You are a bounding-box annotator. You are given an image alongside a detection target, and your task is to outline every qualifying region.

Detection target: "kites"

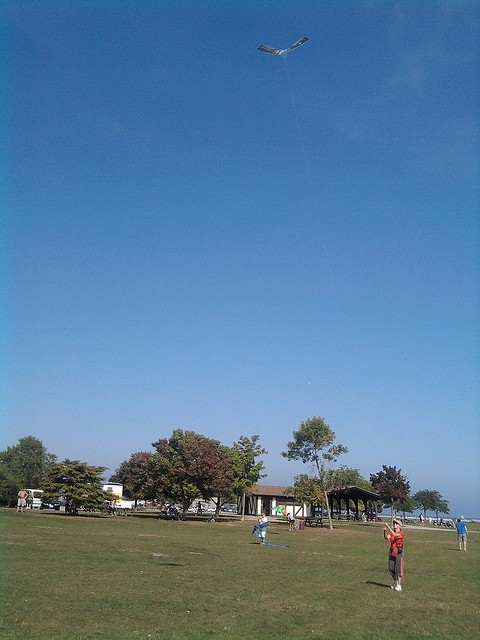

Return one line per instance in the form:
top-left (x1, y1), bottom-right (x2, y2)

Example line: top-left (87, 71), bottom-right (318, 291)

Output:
top-left (256, 37), bottom-right (309, 55)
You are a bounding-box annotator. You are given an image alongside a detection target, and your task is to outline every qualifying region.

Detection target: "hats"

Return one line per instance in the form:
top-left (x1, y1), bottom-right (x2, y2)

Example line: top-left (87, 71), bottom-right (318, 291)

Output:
top-left (393, 518), bottom-right (402, 526)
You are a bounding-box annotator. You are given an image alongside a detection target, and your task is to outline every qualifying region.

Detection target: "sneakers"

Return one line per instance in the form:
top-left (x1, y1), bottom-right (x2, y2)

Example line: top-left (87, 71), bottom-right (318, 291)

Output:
top-left (390, 581), bottom-right (402, 591)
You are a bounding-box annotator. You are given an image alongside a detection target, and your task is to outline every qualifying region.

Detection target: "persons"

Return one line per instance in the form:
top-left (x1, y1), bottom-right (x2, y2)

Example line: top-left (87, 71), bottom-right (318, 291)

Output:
top-left (165, 500), bottom-right (182, 521)
top-left (384, 519), bottom-right (405, 591)
top-left (258, 510), bottom-right (267, 544)
top-left (196, 501), bottom-right (203, 516)
top-left (287, 513), bottom-right (294, 531)
top-left (418, 513), bottom-right (424, 525)
top-left (16, 488), bottom-right (33, 513)
top-left (436, 517), bottom-right (444, 524)
top-left (455, 517), bottom-right (468, 551)
top-left (112, 499), bottom-right (117, 516)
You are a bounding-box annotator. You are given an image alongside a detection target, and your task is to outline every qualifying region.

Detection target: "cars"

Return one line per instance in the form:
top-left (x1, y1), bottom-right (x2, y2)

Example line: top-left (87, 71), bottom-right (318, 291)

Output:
top-left (40, 501), bottom-right (61, 511)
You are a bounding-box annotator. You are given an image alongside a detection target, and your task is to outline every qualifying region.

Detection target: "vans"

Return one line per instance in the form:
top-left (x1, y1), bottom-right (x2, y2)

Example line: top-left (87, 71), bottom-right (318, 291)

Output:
top-left (21, 488), bottom-right (44, 510)
